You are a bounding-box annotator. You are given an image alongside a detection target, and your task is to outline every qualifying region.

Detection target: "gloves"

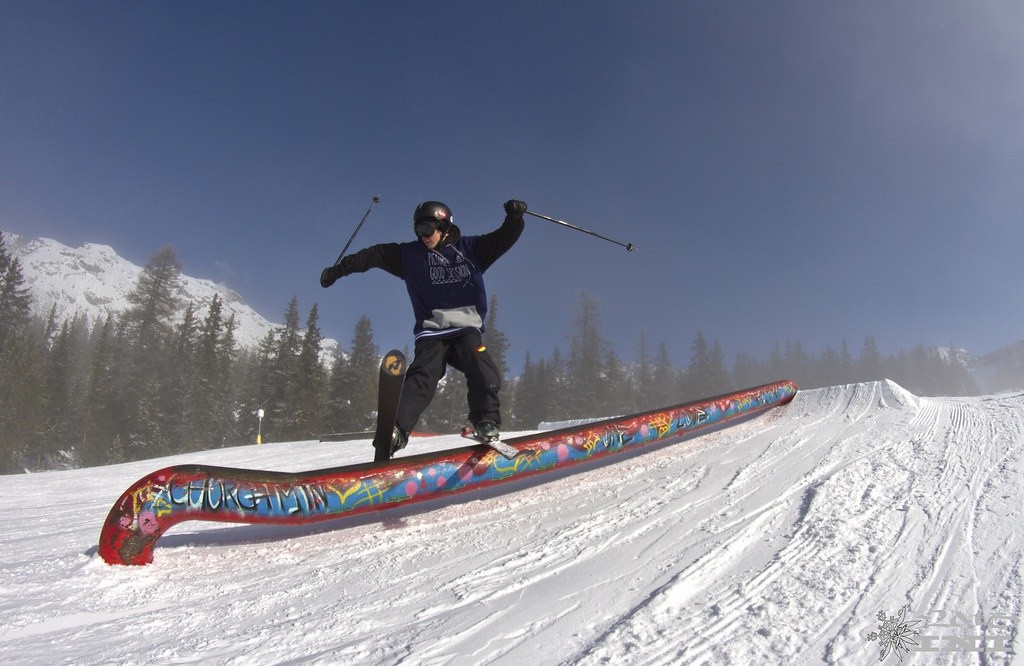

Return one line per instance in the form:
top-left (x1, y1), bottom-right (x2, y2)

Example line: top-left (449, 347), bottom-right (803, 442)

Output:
top-left (320, 266), bottom-right (337, 288)
top-left (505, 199), bottom-right (527, 219)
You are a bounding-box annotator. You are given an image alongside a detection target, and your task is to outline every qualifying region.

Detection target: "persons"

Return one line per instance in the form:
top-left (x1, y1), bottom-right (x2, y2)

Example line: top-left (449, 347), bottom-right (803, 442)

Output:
top-left (320, 198), bottom-right (528, 456)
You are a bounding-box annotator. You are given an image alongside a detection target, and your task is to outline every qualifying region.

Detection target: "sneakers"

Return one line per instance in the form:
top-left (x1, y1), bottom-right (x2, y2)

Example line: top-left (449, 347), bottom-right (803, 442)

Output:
top-left (476, 421), bottom-right (500, 439)
top-left (372, 426), bottom-right (407, 457)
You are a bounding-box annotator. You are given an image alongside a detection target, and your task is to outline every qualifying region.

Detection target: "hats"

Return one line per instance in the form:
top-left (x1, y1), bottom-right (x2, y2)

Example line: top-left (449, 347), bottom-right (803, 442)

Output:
top-left (412, 200), bottom-right (454, 224)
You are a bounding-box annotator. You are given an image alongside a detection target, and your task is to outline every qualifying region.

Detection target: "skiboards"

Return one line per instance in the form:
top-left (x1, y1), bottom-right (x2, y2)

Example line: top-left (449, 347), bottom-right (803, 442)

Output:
top-left (374, 349), bottom-right (408, 462)
top-left (459, 427), bottom-right (520, 460)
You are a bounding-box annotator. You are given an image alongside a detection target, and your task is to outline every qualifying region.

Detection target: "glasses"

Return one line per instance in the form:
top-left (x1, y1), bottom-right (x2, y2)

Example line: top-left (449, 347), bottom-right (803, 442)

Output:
top-left (414, 221), bottom-right (442, 238)
